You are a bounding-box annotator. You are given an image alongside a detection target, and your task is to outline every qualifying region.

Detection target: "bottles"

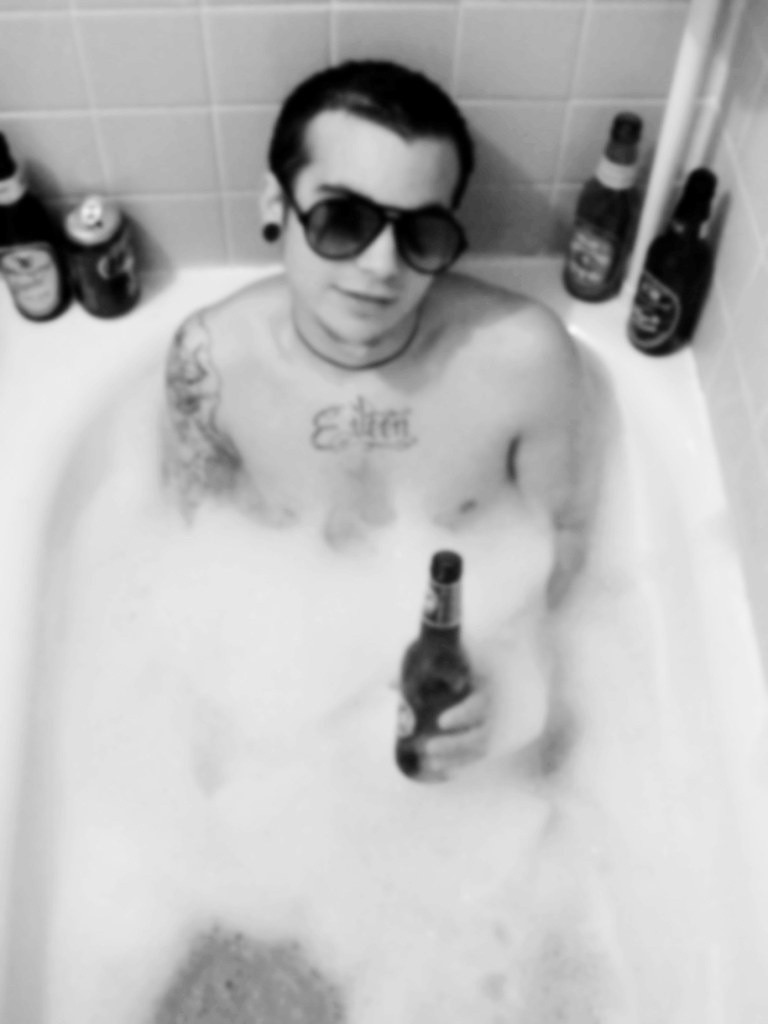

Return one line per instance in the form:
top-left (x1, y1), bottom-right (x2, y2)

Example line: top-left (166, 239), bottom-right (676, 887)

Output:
top-left (627, 166), bottom-right (721, 355)
top-left (398, 549), bottom-right (473, 784)
top-left (562, 110), bottom-right (643, 302)
top-left (0, 130), bottom-right (77, 322)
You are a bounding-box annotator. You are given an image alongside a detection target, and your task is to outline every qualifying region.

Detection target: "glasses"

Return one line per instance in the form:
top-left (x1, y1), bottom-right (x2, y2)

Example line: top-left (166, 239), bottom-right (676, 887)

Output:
top-left (286, 185), bottom-right (468, 274)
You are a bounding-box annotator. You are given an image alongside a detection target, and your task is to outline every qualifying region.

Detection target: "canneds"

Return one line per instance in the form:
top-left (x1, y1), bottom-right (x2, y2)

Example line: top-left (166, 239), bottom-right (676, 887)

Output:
top-left (64, 196), bottom-right (140, 319)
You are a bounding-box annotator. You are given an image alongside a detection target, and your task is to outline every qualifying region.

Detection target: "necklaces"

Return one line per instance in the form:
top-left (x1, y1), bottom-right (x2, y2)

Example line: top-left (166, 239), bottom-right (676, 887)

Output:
top-left (293, 291), bottom-right (424, 371)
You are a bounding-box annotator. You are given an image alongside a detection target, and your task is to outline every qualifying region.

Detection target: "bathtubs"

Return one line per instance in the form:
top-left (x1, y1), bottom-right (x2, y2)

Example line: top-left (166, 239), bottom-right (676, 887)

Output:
top-left (0, 258), bottom-right (767, 1023)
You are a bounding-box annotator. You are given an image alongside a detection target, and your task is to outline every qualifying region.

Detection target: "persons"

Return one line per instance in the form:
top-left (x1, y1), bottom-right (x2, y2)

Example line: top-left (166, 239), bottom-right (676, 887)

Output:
top-left (162, 60), bottom-right (580, 1022)
